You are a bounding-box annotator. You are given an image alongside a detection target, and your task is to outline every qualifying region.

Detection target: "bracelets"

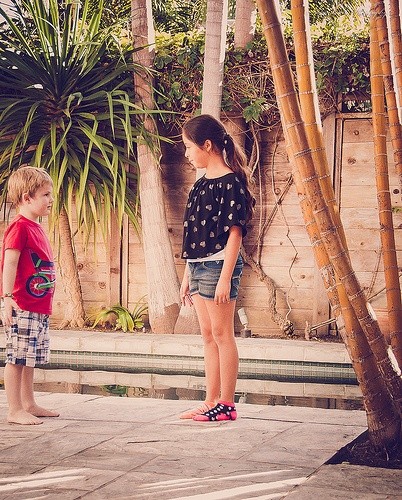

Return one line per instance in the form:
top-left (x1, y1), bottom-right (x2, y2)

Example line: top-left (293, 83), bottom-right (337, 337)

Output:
top-left (2, 292), bottom-right (13, 297)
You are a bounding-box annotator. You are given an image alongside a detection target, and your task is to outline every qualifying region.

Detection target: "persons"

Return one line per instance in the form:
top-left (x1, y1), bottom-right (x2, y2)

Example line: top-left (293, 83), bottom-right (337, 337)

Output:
top-left (176, 114), bottom-right (256, 422)
top-left (0, 164), bottom-right (61, 427)
